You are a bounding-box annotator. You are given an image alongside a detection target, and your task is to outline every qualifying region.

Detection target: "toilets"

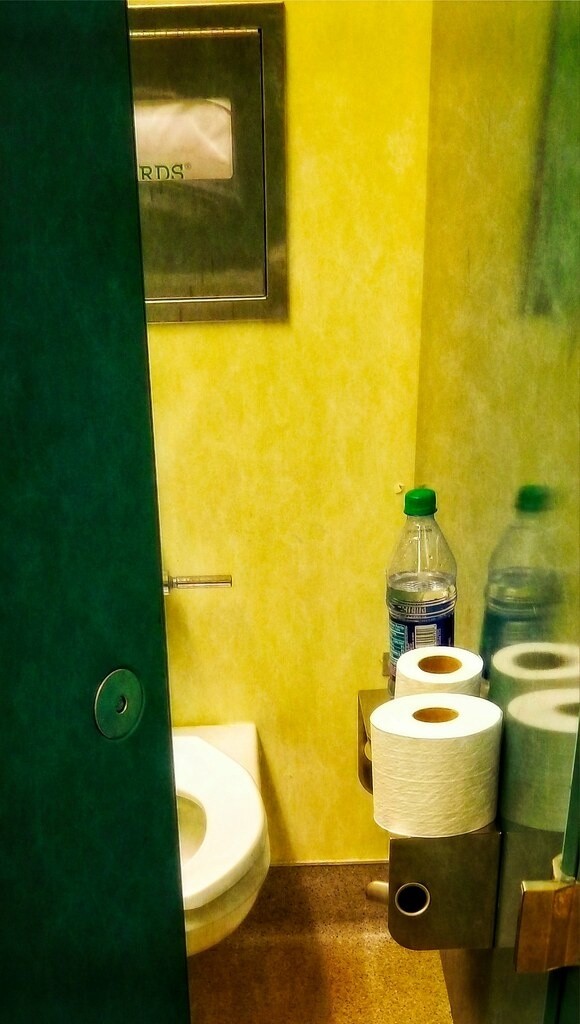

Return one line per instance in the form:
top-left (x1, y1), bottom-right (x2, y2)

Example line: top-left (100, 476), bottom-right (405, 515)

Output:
top-left (173, 721), bottom-right (268, 960)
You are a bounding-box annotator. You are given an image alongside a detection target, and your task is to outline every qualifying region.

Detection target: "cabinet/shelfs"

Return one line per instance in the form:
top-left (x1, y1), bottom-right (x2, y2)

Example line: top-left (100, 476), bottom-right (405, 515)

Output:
top-left (128, 28), bottom-right (267, 302)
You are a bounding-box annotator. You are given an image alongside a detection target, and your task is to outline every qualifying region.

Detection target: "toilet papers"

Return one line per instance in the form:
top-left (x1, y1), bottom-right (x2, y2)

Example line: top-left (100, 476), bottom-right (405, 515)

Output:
top-left (393, 642), bottom-right (486, 706)
top-left (503, 688), bottom-right (580, 833)
top-left (490, 640), bottom-right (580, 711)
top-left (369, 694), bottom-right (502, 840)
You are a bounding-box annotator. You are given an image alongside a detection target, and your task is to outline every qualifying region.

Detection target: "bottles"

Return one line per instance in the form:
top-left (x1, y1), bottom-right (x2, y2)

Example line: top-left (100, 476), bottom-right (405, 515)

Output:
top-left (384, 488), bottom-right (458, 700)
top-left (478, 483), bottom-right (566, 700)
